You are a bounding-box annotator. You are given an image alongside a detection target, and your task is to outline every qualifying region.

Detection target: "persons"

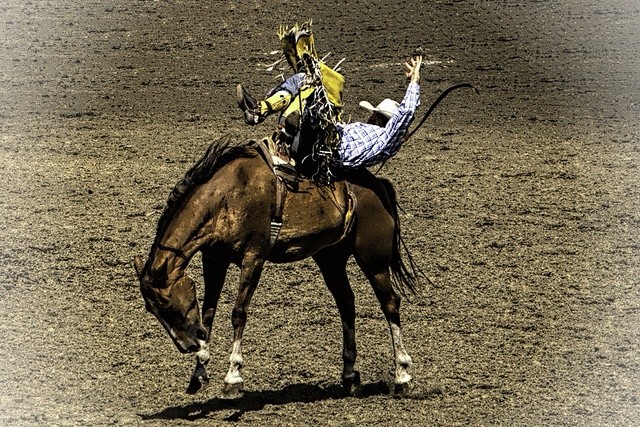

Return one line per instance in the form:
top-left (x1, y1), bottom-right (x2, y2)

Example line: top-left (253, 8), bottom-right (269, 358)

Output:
top-left (236, 53), bottom-right (422, 178)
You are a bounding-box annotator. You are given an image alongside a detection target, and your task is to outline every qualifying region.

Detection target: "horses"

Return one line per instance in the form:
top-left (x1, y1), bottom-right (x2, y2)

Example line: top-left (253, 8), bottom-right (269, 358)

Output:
top-left (134, 132), bottom-right (445, 398)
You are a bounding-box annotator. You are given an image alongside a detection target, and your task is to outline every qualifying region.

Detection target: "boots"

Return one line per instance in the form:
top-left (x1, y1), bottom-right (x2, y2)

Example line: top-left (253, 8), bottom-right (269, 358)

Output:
top-left (278, 86), bottom-right (313, 142)
top-left (237, 84), bottom-right (292, 126)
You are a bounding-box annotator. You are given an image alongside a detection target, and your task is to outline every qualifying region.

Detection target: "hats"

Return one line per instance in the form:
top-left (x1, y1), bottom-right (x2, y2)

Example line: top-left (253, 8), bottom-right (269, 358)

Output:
top-left (359, 99), bottom-right (401, 120)
